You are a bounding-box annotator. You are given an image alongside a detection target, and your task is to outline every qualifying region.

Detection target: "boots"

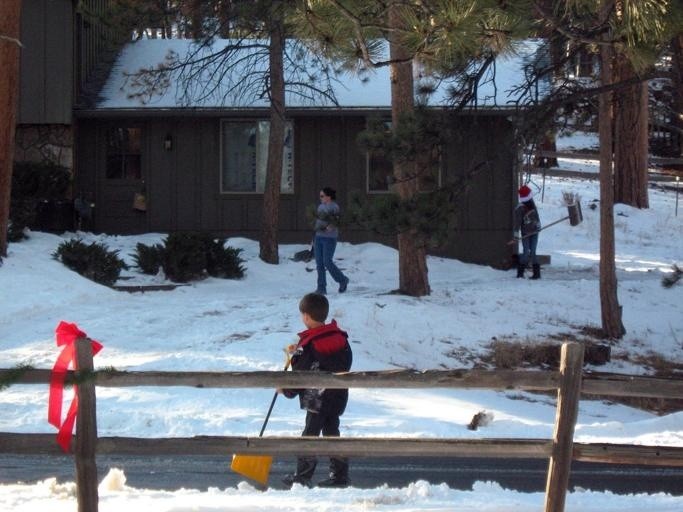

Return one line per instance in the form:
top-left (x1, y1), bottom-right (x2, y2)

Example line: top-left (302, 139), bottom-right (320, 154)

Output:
top-left (529, 263), bottom-right (541, 279)
top-left (517, 263), bottom-right (525, 278)
top-left (319, 454), bottom-right (350, 488)
top-left (282, 456), bottom-right (318, 489)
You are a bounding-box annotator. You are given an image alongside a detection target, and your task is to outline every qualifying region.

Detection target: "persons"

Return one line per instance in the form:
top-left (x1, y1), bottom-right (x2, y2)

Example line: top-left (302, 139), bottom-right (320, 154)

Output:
top-left (509, 183), bottom-right (542, 281)
top-left (306, 186), bottom-right (350, 294)
top-left (274, 291), bottom-right (352, 489)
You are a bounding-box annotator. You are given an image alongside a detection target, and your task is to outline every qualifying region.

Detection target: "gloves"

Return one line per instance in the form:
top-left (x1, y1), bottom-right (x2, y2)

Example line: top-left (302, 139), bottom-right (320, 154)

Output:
top-left (514, 232), bottom-right (519, 240)
top-left (536, 222), bottom-right (540, 231)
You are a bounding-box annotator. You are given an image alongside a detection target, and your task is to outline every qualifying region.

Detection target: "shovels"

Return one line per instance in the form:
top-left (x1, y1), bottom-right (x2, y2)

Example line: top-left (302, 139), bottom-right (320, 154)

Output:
top-left (507, 201), bottom-right (583, 246)
top-left (230, 345), bottom-right (297, 485)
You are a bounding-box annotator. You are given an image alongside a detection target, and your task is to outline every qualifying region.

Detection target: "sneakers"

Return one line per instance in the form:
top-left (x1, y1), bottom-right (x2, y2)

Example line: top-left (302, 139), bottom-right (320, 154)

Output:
top-left (313, 289), bottom-right (327, 295)
top-left (339, 277), bottom-right (348, 293)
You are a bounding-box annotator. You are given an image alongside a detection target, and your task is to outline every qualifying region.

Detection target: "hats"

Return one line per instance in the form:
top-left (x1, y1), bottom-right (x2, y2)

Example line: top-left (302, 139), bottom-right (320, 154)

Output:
top-left (518, 186), bottom-right (532, 202)
